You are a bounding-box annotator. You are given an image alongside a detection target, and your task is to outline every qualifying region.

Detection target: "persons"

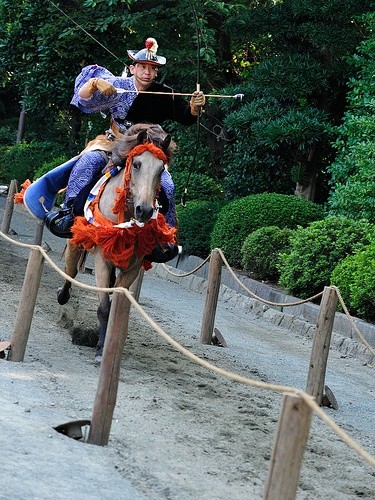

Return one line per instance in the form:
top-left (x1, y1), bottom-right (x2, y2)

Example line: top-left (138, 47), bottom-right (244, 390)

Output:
top-left (53, 38), bottom-right (205, 228)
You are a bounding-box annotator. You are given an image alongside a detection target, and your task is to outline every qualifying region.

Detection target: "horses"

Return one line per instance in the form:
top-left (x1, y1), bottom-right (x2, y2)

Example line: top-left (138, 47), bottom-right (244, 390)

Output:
top-left (56, 121), bottom-right (175, 366)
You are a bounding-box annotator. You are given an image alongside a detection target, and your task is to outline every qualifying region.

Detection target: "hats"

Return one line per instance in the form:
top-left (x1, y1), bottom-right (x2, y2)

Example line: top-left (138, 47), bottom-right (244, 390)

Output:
top-left (126, 38), bottom-right (168, 66)
